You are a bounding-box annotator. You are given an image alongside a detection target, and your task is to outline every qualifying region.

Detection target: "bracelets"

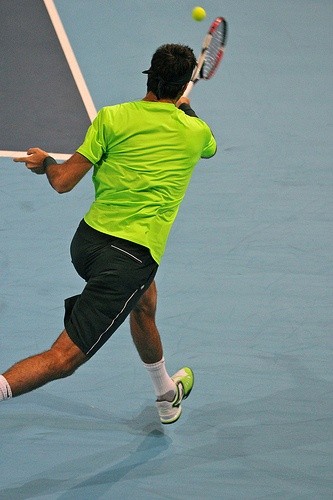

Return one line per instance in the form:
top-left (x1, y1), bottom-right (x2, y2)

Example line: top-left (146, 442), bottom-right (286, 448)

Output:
top-left (42, 157), bottom-right (56, 171)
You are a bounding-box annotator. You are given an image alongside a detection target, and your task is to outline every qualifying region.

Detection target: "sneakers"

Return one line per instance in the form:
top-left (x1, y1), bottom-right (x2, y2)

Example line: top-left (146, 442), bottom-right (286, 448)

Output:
top-left (155, 367), bottom-right (194, 425)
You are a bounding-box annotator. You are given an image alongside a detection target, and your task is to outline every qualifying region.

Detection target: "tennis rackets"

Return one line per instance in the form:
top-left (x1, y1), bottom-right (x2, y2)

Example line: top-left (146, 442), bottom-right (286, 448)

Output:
top-left (182, 16), bottom-right (228, 97)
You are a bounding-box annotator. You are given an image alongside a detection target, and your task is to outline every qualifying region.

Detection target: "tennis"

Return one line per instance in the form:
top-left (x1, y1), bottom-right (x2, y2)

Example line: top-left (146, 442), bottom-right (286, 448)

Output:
top-left (191, 6), bottom-right (207, 22)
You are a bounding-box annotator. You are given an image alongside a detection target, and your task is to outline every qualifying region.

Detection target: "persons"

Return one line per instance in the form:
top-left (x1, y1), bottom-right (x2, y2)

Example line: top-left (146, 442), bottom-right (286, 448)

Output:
top-left (0, 43), bottom-right (218, 425)
